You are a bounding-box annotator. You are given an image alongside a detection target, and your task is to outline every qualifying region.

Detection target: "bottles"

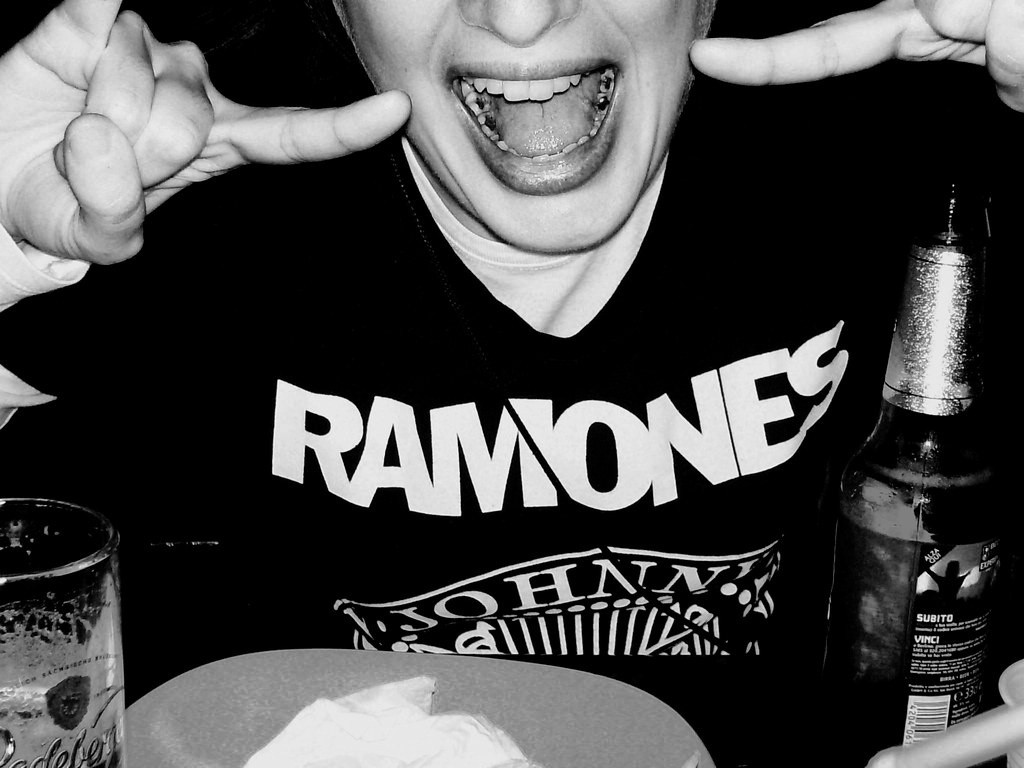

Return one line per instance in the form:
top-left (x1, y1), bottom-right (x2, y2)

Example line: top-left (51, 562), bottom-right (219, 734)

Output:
top-left (817, 173), bottom-right (1002, 768)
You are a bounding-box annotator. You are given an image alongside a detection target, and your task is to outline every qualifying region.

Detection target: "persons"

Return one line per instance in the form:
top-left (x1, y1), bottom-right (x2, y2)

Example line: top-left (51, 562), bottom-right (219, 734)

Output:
top-left (0, 0), bottom-right (1024, 656)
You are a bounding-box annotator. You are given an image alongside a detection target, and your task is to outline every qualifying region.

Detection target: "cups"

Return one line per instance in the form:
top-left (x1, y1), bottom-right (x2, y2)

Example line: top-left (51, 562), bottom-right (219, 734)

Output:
top-left (0, 499), bottom-right (128, 768)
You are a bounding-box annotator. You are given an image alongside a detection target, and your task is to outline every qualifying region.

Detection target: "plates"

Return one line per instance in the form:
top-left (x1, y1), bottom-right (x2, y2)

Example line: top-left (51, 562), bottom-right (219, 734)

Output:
top-left (121, 649), bottom-right (715, 768)
